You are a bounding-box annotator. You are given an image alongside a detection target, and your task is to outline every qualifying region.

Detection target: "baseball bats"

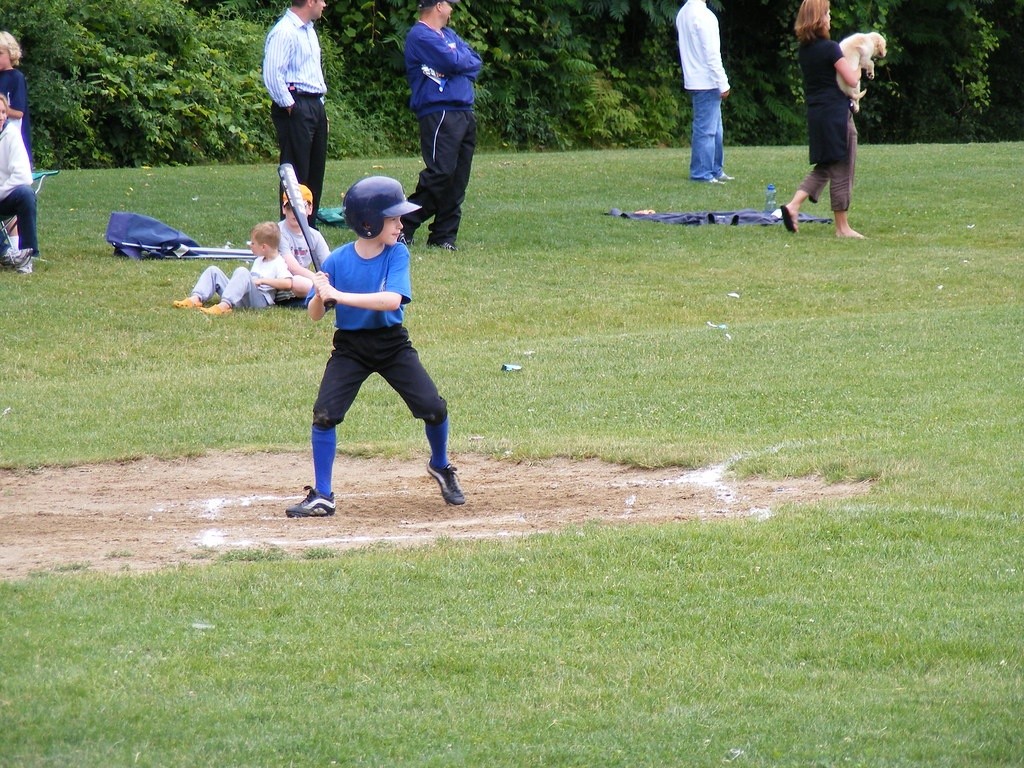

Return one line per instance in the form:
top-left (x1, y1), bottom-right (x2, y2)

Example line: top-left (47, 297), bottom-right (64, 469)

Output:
top-left (277, 163), bottom-right (337, 308)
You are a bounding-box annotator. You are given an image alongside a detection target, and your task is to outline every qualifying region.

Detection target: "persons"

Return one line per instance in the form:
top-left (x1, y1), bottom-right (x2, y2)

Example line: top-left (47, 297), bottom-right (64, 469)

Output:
top-left (0, 92), bottom-right (39, 274)
top-left (0, 31), bottom-right (31, 251)
top-left (780, 0), bottom-right (864, 238)
top-left (263, 0), bottom-right (327, 229)
top-left (285, 176), bottom-right (465, 518)
top-left (173, 221), bottom-right (293, 315)
top-left (397, 0), bottom-right (482, 251)
top-left (676, 0), bottom-right (735, 185)
top-left (0, 233), bottom-right (33, 267)
top-left (277, 184), bottom-right (331, 307)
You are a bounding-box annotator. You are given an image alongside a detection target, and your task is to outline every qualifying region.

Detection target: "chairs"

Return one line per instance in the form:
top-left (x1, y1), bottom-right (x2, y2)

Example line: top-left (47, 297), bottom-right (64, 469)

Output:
top-left (0, 170), bottom-right (60, 251)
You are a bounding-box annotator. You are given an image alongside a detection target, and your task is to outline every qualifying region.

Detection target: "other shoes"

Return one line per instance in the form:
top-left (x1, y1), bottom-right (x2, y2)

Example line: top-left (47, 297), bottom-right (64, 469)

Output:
top-left (398, 230), bottom-right (415, 247)
top-left (438, 240), bottom-right (458, 252)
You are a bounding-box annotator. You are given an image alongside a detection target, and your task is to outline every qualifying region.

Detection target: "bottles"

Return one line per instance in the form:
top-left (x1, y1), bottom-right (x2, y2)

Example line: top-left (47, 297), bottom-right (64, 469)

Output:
top-left (764, 183), bottom-right (777, 213)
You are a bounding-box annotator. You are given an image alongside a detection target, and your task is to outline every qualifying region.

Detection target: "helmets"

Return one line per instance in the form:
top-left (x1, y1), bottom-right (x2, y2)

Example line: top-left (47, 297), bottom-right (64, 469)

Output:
top-left (343, 175), bottom-right (423, 239)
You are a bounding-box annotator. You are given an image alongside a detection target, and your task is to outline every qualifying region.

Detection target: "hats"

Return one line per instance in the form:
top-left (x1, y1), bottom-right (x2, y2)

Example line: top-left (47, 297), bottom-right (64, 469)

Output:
top-left (282, 184), bottom-right (313, 207)
top-left (417, 0), bottom-right (460, 8)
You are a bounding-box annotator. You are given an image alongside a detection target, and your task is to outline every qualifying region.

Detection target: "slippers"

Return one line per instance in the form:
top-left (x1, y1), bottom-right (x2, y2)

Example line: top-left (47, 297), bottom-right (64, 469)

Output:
top-left (779, 205), bottom-right (800, 233)
top-left (198, 304), bottom-right (232, 318)
top-left (172, 298), bottom-right (204, 310)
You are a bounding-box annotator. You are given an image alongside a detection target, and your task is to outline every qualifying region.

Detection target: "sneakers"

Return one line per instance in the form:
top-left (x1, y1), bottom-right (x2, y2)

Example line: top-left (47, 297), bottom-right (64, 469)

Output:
top-left (709, 177), bottom-right (725, 184)
top-left (719, 171), bottom-right (735, 182)
top-left (17, 259), bottom-right (32, 273)
top-left (427, 456), bottom-right (466, 506)
top-left (0, 247), bottom-right (33, 268)
top-left (286, 486), bottom-right (336, 518)
